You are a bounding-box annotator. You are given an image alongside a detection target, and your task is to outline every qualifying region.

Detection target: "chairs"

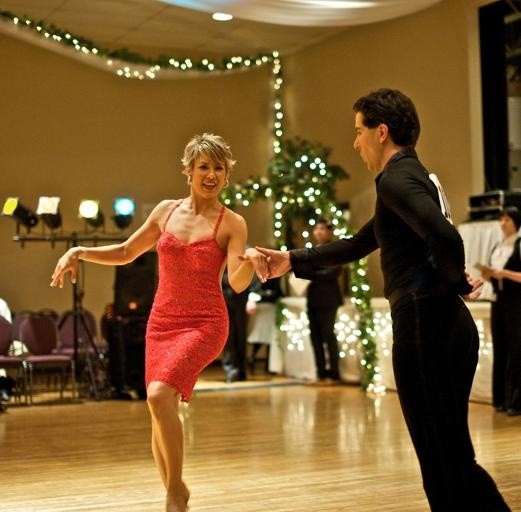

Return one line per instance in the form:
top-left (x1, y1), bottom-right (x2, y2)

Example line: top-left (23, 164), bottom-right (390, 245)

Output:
top-left (0, 309), bottom-right (107, 405)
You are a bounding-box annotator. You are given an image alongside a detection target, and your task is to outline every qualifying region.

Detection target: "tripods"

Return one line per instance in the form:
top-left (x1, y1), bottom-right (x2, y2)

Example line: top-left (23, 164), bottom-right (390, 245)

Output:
top-left (54, 239), bottom-right (113, 401)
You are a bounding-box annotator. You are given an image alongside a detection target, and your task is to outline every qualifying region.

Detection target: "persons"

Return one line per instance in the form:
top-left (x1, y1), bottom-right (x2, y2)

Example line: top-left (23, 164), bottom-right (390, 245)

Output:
top-left (246, 85), bottom-right (513, 511)
top-left (99, 297), bottom-right (147, 401)
top-left (298, 215), bottom-right (344, 382)
top-left (48, 128), bottom-right (272, 512)
top-left (219, 266), bottom-right (285, 383)
top-left (477, 205), bottom-right (521, 415)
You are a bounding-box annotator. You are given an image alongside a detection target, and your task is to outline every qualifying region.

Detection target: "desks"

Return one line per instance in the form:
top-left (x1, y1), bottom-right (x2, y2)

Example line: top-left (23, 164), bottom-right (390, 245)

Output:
top-left (278, 294), bottom-right (492, 404)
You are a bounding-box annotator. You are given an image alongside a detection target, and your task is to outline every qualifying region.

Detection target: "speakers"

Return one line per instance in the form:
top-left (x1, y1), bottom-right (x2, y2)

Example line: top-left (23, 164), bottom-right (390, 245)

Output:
top-left (114, 251), bottom-right (159, 400)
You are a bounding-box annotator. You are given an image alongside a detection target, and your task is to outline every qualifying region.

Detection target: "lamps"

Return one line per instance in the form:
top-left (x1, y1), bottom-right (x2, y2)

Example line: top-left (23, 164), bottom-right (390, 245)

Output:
top-left (0, 194), bottom-right (138, 399)
